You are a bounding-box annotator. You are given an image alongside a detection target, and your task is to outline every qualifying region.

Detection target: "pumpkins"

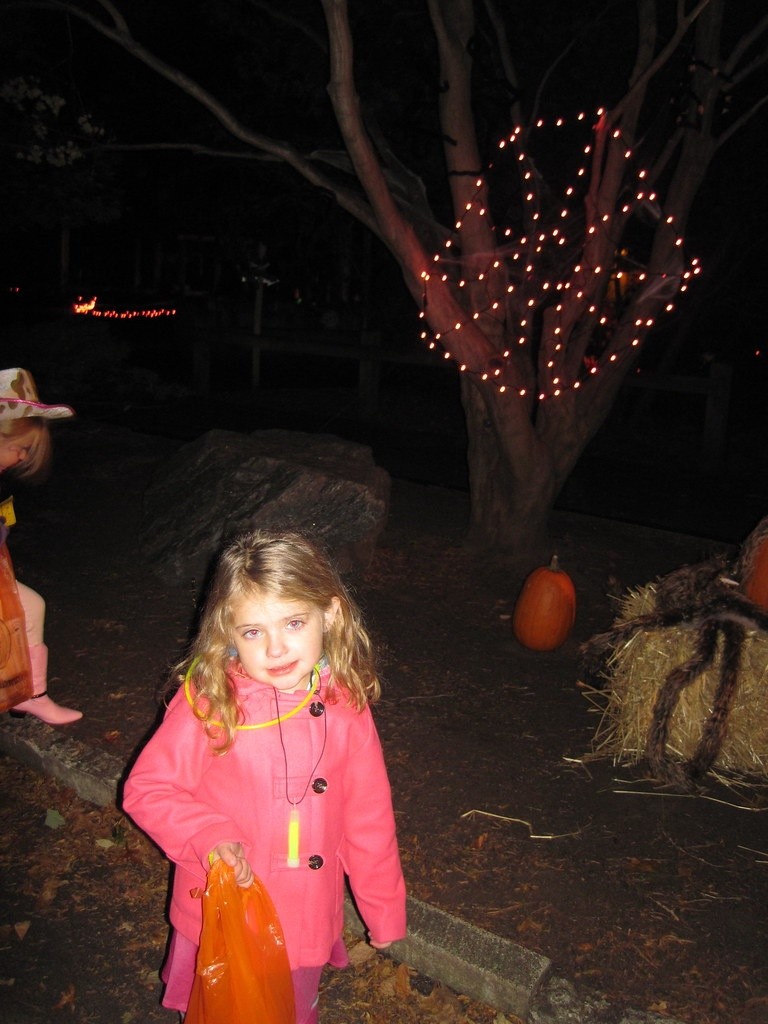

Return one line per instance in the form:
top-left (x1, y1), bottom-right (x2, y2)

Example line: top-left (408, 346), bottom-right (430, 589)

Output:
top-left (514, 554), bottom-right (576, 652)
top-left (740, 534), bottom-right (768, 618)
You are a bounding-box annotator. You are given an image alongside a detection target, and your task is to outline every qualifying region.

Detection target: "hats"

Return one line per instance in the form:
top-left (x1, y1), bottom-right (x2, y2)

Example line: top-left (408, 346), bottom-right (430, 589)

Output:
top-left (0, 368), bottom-right (76, 422)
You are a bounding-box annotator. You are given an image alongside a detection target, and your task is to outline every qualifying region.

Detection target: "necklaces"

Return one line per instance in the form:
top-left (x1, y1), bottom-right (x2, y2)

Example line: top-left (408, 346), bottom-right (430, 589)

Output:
top-left (184, 652), bottom-right (328, 872)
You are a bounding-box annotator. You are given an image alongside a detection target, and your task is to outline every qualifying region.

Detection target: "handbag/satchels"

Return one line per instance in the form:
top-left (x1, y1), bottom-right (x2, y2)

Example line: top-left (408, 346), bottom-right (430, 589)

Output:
top-left (184, 858), bottom-right (298, 1024)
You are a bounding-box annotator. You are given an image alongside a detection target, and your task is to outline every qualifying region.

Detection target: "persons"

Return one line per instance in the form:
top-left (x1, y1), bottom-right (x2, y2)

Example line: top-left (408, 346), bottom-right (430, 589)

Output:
top-left (0, 367), bottom-right (83, 722)
top-left (121, 528), bottom-right (408, 1024)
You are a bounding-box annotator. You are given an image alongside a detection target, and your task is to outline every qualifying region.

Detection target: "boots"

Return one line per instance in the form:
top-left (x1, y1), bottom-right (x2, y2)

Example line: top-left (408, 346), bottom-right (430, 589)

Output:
top-left (8, 644), bottom-right (84, 725)
